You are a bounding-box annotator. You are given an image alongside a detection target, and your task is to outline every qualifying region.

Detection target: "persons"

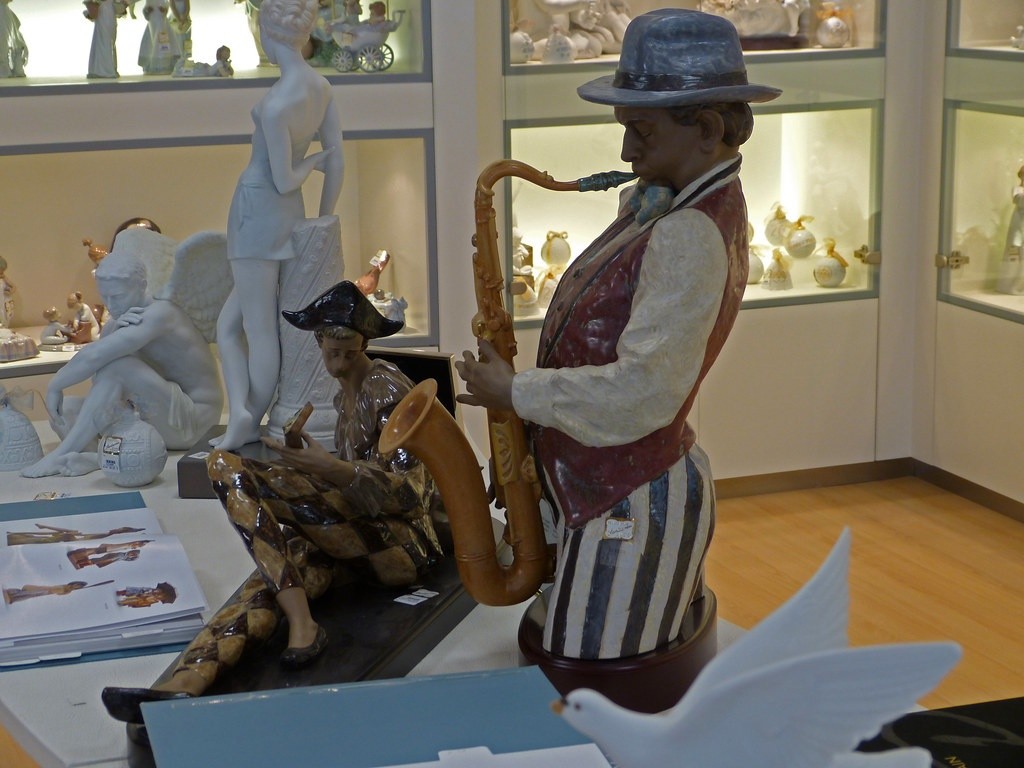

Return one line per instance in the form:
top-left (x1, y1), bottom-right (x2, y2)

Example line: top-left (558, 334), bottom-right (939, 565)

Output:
top-left (326, 1), bottom-right (398, 34)
top-left (171, 46), bottom-right (234, 77)
top-left (138, 0), bottom-right (191, 75)
top-left (455, 10), bottom-right (784, 660)
top-left (21, 252), bottom-right (224, 478)
top-left (40, 291), bottom-right (100, 345)
top-left (101, 280), bottom-right (446, 725)
top-left (208, 0), bottom-right (344, 453)
top-left (83, 0), bottom-right (136, 78)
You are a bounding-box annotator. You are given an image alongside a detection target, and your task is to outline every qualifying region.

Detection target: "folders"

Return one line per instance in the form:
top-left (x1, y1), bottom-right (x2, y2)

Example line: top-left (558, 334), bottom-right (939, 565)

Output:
top-left (139, 663), bottom-right (611, 768)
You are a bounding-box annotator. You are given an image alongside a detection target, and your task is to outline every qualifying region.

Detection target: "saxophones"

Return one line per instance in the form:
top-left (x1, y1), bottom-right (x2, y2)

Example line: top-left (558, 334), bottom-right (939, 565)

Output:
top-left (378, 160), bottom-right (640, 607)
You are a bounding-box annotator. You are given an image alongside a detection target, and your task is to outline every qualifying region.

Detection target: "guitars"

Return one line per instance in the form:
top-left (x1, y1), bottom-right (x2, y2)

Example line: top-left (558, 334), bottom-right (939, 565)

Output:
top-left (283, 400), bottom-right (315, 449)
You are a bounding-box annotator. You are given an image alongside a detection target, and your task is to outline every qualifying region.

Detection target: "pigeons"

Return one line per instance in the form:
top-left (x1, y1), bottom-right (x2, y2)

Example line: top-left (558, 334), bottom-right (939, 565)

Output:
top-left (551, 526), bottom-right (965, 768)
